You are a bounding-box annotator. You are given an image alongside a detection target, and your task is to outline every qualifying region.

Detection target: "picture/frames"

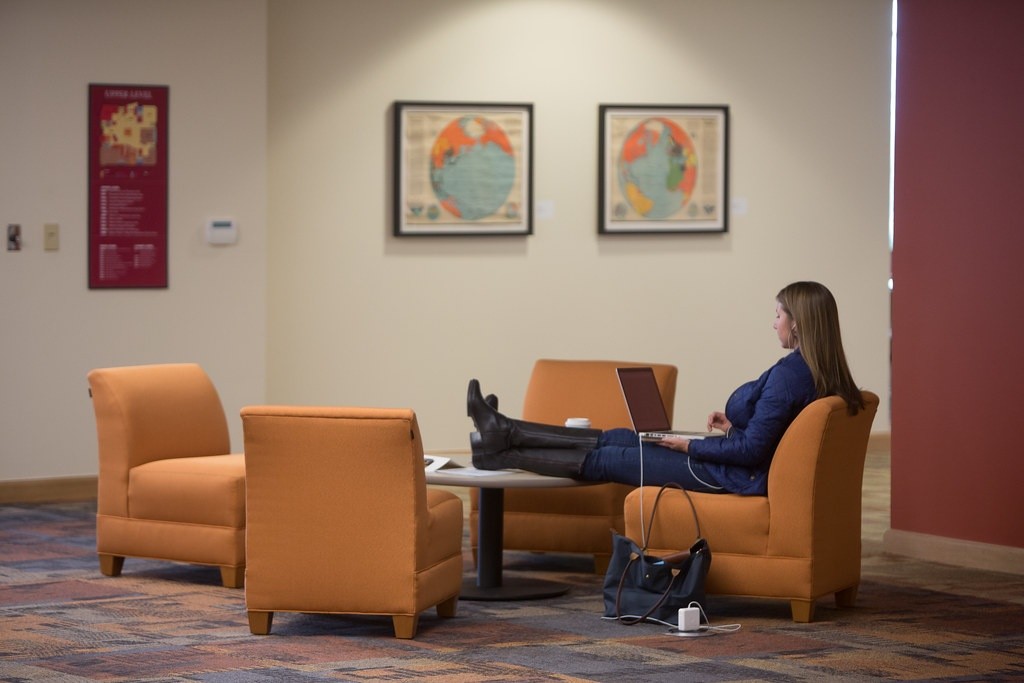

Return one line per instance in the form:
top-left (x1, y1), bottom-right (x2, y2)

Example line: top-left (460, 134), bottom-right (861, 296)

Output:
top-left (393, 101), bottom-right (533, 235)
top-left (598, 104), bottom-right (729, 233)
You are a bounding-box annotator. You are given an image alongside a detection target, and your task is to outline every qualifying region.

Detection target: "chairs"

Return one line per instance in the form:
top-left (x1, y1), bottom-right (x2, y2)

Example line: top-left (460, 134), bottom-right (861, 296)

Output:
top-left (238, 406), bottom-right (463, 639)
top-left (470, 358), bottom-right (678, 576)
top-left (624, 390), bottom-right (879, 622)
top-left (88, 363), bottom-right (246, 589)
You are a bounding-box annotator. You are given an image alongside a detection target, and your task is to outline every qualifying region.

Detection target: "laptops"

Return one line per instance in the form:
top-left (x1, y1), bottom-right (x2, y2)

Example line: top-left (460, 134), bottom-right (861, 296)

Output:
top-left (616, 367), bottom-right (726, 442)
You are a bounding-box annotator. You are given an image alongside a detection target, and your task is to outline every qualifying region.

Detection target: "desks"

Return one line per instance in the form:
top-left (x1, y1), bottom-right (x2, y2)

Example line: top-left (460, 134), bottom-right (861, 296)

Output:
top-left (425, 454), bottom-right (619, 601)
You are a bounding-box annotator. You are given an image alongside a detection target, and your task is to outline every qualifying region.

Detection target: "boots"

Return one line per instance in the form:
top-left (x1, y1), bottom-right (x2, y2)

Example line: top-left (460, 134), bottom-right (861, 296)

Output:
top-left (472, 394), bottom-right (591, 480)
top-left (466, 379), bottom-right (602, 455)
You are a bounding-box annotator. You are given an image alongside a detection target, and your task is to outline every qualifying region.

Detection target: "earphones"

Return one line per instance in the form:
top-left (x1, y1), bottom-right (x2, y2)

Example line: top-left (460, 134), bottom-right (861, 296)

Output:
top-left (790, 323), bottom-right (796, 331)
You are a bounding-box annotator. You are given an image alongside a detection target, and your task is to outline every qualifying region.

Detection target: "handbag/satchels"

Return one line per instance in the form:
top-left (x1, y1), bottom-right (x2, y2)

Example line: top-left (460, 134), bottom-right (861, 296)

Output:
top-left (601, 482), bottom-right (711, 625)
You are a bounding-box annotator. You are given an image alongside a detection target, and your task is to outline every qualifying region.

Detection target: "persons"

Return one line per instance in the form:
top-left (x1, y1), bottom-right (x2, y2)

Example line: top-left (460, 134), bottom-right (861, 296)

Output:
top-left (467, 281), bottom-right (865, 497)
